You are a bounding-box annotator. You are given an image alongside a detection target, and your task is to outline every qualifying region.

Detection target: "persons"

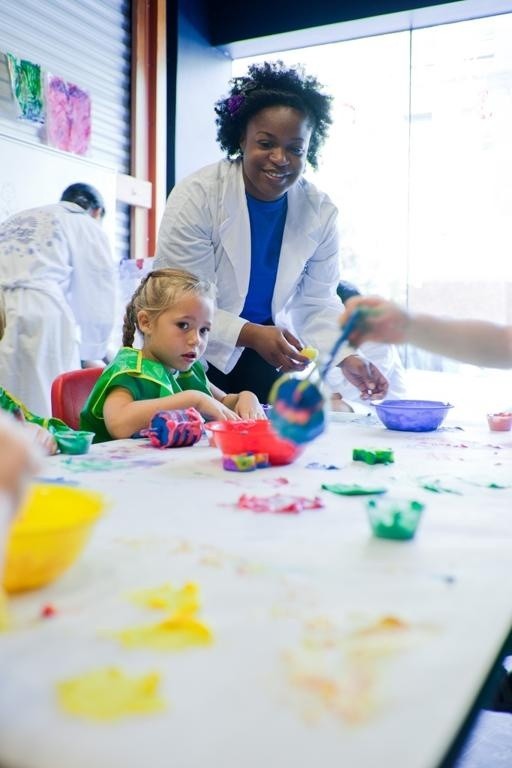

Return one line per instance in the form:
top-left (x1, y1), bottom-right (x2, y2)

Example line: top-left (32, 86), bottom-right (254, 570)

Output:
top-left (337, 294), bottom-right (512, 369)
top-left (0, 306), bottom-right (71, 456)
top-left (152, 61), bottom-right (389, 401)
top-left (0, 182), bottom-right (124, 420)
top-left (78, 267), bottom-right (268, 444)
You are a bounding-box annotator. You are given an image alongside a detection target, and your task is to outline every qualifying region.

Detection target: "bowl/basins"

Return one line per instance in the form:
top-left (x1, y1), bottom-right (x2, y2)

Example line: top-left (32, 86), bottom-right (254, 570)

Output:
top-left (54, 431), bottom-right (96, 455)
top-left (0, 483), bottom-right (103, 594)
top-left (370, 400), bottom-right (455, 432)
top-left (204, 420), bottom-right (306, 466)
top-left (487, 412), bottom-right (512, 431)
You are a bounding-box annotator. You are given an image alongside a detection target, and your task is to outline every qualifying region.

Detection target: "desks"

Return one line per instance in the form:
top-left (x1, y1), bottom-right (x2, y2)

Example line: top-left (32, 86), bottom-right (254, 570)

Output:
top-left (1, 405), bottom-right (512, 768)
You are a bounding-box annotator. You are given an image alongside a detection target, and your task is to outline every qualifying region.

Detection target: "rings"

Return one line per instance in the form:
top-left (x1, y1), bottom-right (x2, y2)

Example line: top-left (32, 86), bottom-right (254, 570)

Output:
top-left (276, 365), bottom-right (283, 373)
